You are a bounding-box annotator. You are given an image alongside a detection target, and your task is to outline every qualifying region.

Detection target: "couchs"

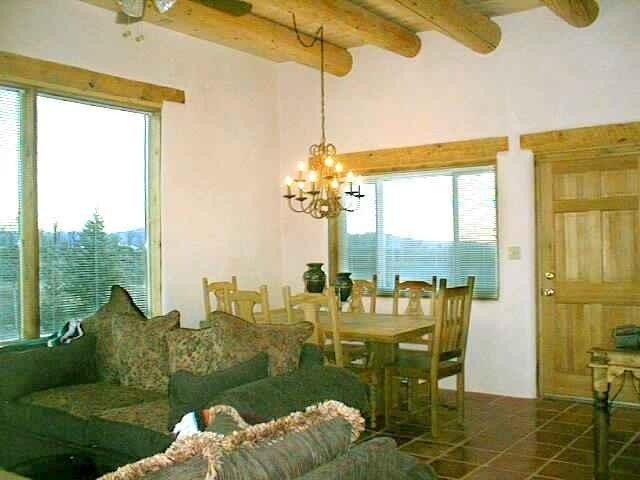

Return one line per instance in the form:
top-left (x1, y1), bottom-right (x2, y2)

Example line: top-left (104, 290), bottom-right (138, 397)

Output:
top-left (1, 331), bottom-right (415, 480)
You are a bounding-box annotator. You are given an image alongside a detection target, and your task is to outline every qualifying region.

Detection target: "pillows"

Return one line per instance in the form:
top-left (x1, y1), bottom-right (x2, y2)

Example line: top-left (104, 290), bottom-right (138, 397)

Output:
top-left (78, 285), bottom-right (364, 480)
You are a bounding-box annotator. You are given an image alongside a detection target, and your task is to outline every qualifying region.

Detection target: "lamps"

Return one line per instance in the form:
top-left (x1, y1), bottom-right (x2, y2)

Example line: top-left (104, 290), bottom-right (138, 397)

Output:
top-left (281, 11), bottom-right (367, 221)
top-left (112, 1), bottom-right (176, 45)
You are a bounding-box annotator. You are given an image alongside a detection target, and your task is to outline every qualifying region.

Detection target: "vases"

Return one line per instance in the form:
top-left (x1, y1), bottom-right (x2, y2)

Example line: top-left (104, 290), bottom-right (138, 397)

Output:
top-left (335, 272), bottom-right (353, 301)
top-left (303, 259), bottom-right (327, 292)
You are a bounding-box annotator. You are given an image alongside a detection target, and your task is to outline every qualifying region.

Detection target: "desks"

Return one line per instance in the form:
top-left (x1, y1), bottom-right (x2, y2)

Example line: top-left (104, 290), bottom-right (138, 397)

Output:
top-left (585, 345), bottom-right (640, 479)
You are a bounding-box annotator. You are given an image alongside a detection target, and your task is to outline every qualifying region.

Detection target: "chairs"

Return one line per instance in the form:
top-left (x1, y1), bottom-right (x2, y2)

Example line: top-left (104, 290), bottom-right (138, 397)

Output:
top-left (203, 275), bottom-right (476, 440)
